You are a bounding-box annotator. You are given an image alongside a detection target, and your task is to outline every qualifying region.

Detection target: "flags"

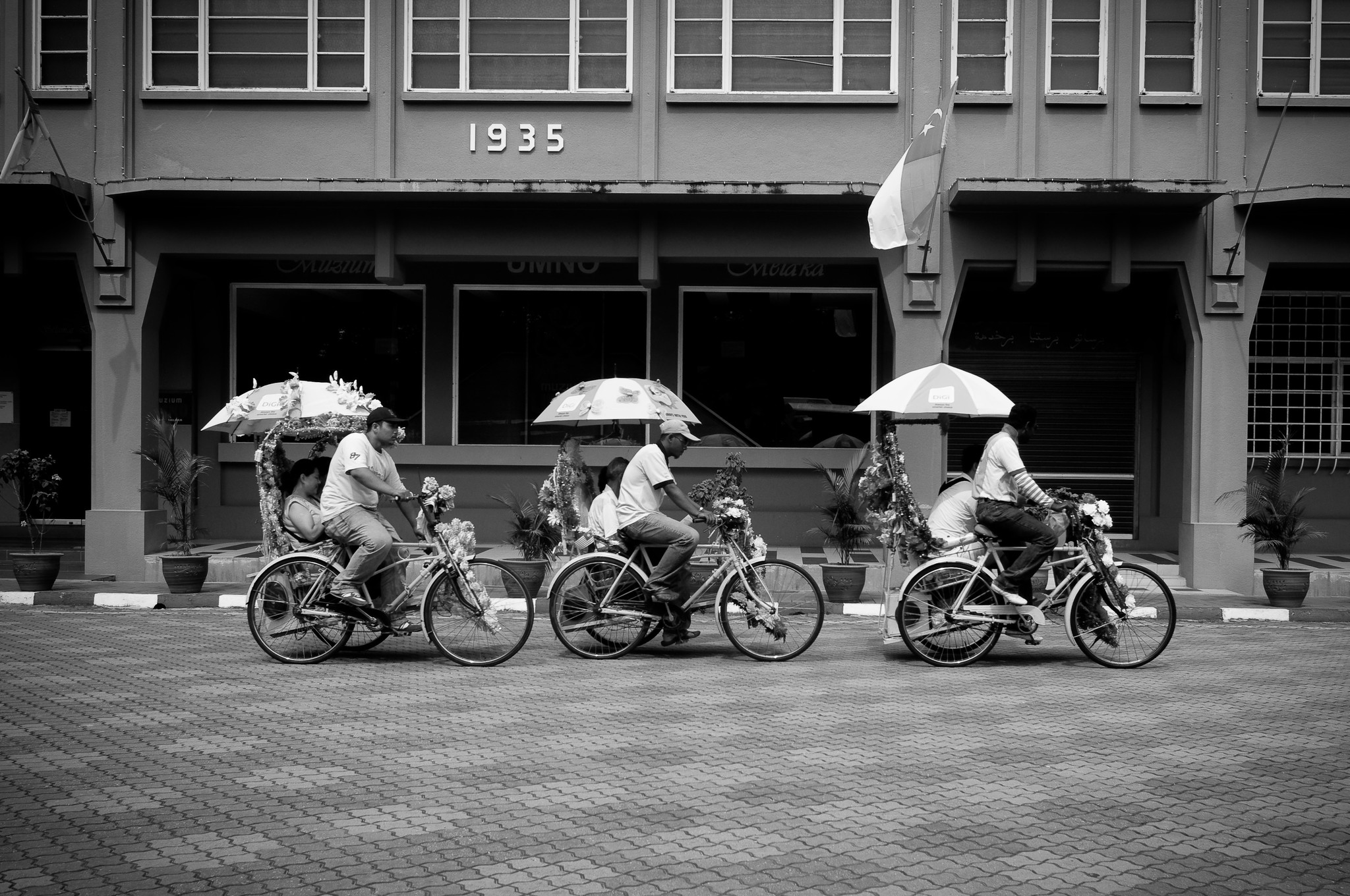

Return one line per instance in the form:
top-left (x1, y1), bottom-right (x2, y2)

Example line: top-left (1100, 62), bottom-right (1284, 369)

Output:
top-left (867, 77), bottom-right (960, 250)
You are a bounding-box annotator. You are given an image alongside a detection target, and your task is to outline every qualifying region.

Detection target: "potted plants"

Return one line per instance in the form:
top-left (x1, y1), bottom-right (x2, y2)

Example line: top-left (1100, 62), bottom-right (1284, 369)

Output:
top-left (127, 409), bottom-right (214, 593)
top-left (801, 439), bottom-right (877, 603)
top-left (0, 447), bottom-right (64, 592)
top-left (485, 481), bottom-right (567, 599)
top-left (1212, 431), bottom-right (1329, 607)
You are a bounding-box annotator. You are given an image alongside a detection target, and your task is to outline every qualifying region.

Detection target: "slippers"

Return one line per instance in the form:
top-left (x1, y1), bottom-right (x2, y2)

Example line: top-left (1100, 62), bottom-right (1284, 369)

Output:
top-left (661, 631), bottom-right (700, 647)
top-left (643, 585), bottom-right (680, 602)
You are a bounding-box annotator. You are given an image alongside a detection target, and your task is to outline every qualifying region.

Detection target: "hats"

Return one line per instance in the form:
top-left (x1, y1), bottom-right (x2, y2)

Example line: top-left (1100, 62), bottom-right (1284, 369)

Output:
top-left (366, 407), bottom-right (410, 426)
top-left (660, 420), bottom-right (701, 443)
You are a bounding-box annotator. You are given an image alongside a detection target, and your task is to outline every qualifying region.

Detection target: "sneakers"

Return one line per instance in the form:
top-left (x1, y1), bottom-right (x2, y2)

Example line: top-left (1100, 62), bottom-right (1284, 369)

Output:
top-left (330, 591), bottom-right (368, 606)
top-left (1006, 630), bottom-right (1043, 641)
top-left (381, 620), bottom-right (423, 634)
top-left (991, 582), bottom-right (1028, 605)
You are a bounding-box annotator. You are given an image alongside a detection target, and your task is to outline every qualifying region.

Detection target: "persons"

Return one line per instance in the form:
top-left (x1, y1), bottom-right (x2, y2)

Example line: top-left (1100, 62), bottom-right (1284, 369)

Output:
top-left (925, 441), bottom-right (1011, 577)
top-left (587, 456), bottom-right (630, 578)
top-left (310, 455), bottom-right (333, 499)
top-left (970, 401), bottom-right (1078, 643)
top-left (615, 419), bottom-right (719, 647)
top-left (320, 407), bottom-right (427, 633)
top-left (280, 458), bottom-right (347, 575)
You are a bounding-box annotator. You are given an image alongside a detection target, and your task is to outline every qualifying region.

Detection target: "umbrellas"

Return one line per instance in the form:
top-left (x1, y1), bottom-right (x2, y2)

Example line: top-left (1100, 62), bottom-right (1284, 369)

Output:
top-left (529, 362), bottom-right (702, 428)
top-left (852, 350), bottom-right (1017, 419)
top-left (200, 367), bottom-right (384, 439)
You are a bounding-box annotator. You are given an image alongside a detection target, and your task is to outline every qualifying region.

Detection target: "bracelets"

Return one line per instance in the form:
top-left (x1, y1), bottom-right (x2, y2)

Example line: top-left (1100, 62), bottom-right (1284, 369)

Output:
top-left (698, 506), bottom-right (704, 512)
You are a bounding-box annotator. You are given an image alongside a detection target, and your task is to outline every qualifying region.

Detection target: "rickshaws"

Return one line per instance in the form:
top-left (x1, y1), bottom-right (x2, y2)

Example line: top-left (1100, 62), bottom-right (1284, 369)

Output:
top-left (530, 376), bottom-right (826, 662)
top-left (851, 364), bottom-right (1177, 668)
top-left (201, 379), bottom-right (535, 668)
